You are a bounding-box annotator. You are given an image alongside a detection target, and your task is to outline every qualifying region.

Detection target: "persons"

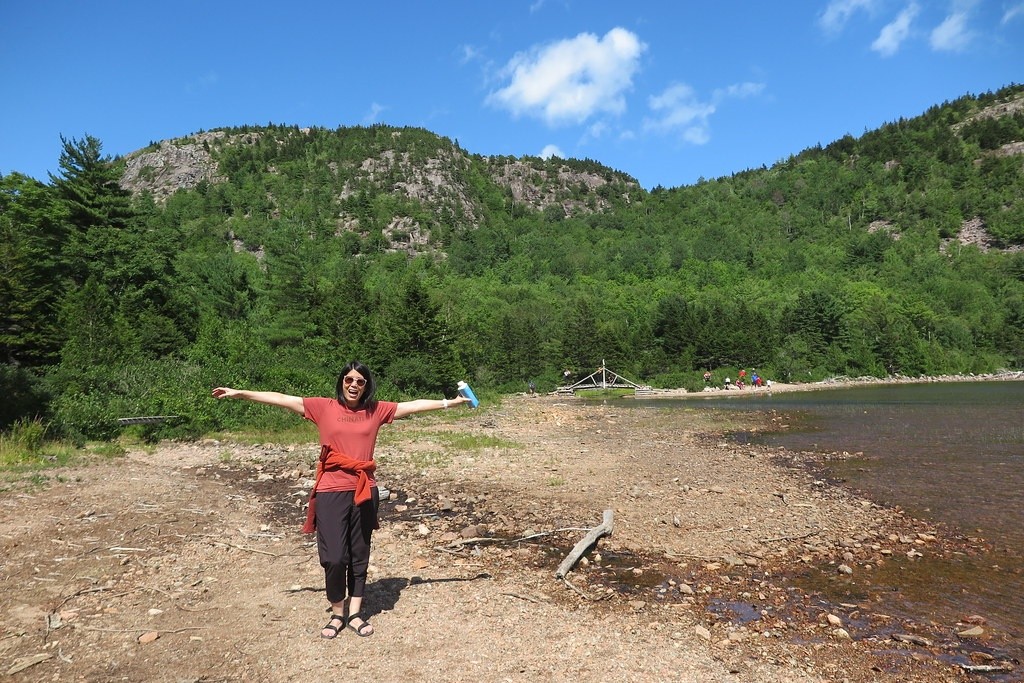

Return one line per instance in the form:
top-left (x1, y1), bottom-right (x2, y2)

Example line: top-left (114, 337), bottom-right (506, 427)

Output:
top-left (704, 371), bottom-right (711, 381)
top-left (563, 369), bottom-right (571, 382)
top-left (530, 380), bottom-right (536, 397)
top-left (608, 374), bottom-right (615, 385)
top-left (211, 361), bottom-right (472, 640)
top-left (724, 366), bottom-right (762, 391)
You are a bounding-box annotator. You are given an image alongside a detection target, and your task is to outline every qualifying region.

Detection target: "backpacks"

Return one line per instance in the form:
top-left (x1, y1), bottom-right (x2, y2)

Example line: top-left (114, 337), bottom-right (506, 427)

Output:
top-left (531, 382), bottom-right (535, 389)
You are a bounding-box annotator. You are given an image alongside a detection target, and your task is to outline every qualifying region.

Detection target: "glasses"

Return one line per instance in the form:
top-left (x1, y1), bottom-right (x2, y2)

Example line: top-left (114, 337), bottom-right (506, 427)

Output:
top-left (344, 375), bottom-right (369, 387)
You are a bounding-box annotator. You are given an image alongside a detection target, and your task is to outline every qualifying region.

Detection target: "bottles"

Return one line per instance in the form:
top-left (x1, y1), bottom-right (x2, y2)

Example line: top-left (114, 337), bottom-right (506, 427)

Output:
top-left (456, 381), bottom-right (479, 408)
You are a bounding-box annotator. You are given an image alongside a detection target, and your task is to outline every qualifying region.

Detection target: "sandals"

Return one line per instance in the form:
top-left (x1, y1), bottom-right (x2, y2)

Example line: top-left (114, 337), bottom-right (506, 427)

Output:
top-left (321, 614), bottom-right (345, 639)
top-left (346, 613), bottom-right (374, 637)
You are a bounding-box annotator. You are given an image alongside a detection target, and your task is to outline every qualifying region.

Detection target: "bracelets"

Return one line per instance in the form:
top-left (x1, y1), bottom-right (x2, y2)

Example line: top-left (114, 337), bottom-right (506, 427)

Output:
top-left (442, 399), bottom-right (448, 410)
